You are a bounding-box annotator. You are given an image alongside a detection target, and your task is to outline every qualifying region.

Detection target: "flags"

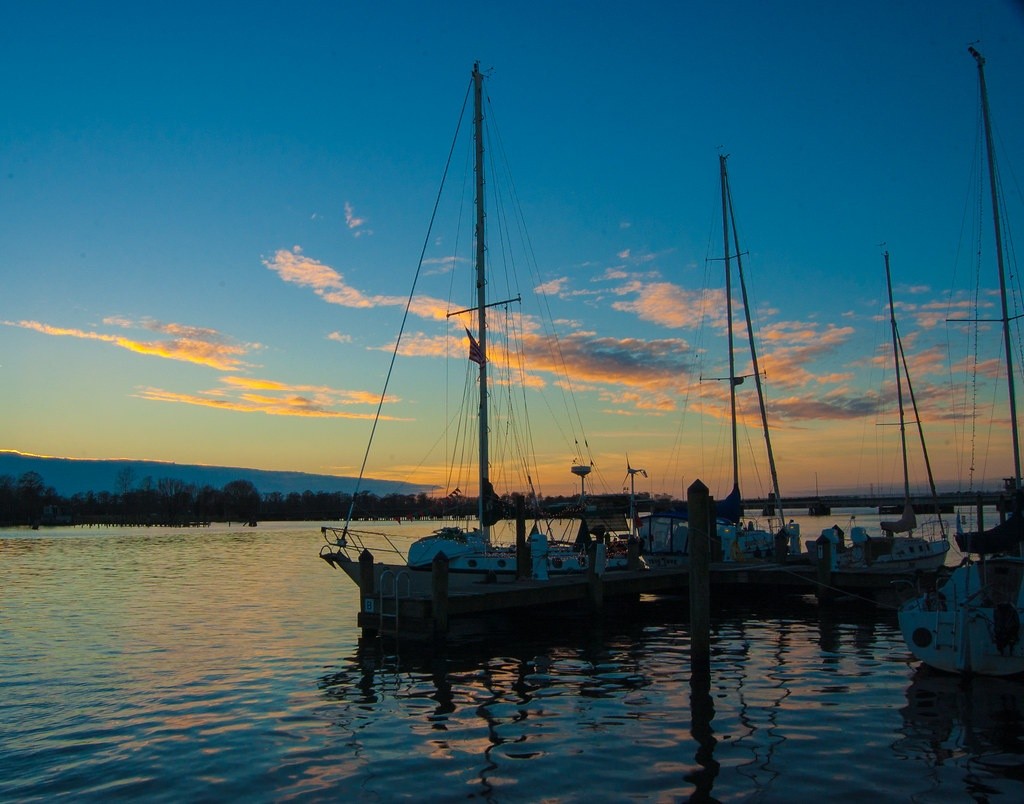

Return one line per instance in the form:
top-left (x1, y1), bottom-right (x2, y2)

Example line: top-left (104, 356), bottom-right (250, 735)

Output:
top-left (465, 326), bottom-right (486, 364)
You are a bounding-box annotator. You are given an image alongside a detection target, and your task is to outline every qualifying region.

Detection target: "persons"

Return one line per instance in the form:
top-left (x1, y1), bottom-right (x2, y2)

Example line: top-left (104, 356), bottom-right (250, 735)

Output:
top-left (609, 537), bottom-right (645, 556)
top-left (775, 527), bottom-right (791, 557)
top-left (925, 585), bottom-right (947, 611)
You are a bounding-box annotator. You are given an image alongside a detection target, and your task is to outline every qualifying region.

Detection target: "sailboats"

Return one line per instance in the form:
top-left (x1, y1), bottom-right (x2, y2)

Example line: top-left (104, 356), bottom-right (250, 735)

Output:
top-left (320, 59), bottom-right (645, 636)
top-left (645, 149), bottom-right (813, 598)
top-left (899, 41), bottom-right (1024, 677)
top-left (806, 253), bottom-right (951, 583)
